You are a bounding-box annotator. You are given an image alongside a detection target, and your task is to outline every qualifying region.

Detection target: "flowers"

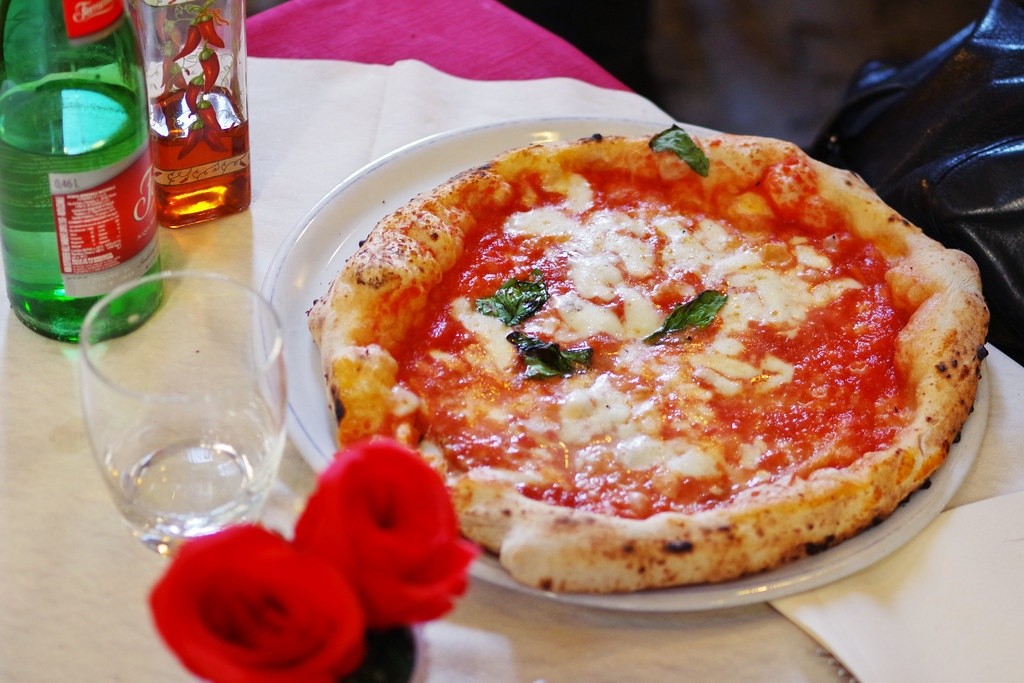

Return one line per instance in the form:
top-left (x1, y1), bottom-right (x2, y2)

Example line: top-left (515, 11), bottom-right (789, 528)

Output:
top-left (146, 433), bottom-right (484, 681)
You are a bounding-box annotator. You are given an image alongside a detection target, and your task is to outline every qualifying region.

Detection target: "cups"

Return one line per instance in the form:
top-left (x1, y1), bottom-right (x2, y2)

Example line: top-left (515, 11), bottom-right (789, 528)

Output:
top-left (80, 269), bottom-right (292, 558)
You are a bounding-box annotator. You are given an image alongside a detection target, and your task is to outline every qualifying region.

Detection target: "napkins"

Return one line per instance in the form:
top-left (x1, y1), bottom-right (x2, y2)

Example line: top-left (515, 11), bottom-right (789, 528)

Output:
top-left (762, 482), bottom-right (1023, 683)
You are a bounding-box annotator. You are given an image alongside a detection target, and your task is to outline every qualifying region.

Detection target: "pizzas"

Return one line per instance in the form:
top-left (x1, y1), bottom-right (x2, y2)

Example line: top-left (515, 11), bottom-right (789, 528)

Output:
top-left (319, 126), bottom-right (991, 598)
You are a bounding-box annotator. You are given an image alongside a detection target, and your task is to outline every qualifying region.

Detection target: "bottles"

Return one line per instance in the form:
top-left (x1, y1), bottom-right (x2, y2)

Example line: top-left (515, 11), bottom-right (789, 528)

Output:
top-left (121, 0), bottom-right (252, 230)
top-left (3, 0), bottom-right (162, 348)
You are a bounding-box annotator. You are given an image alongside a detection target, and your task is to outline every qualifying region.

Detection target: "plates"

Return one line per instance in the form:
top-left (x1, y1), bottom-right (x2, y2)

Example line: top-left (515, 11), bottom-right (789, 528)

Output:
top-left (256, 113), bottom-right (988, 612)
